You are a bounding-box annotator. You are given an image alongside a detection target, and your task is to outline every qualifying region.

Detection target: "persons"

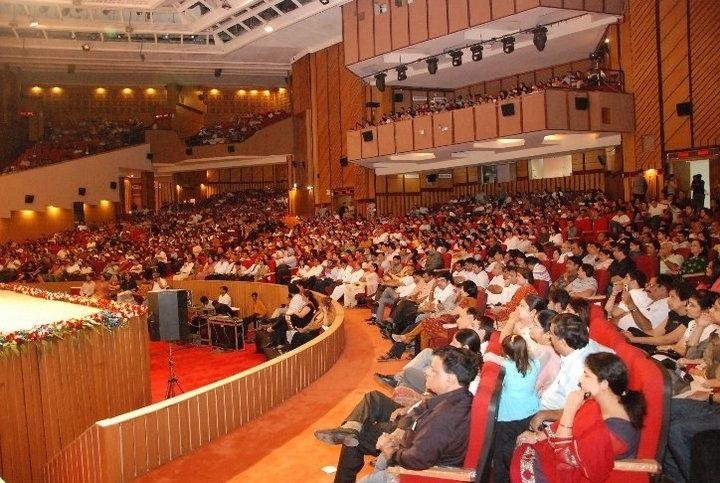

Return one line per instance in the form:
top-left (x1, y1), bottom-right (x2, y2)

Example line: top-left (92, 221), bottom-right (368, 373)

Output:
top-left (1, 168), bottom-right (720, 483)
top-left (0, 106), bottom-right (296, 167)
top-left (347, 49), bottom-right (618, 133)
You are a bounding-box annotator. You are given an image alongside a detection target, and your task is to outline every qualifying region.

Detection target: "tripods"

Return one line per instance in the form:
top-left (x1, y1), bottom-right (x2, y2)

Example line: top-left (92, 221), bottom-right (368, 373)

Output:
top-left (164, 342), bottom-right (184, 399)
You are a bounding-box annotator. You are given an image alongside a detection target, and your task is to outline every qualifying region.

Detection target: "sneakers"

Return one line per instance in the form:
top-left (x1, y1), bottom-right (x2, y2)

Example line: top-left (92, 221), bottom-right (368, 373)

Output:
top-left (264, 343), bottom-right (288, 350)
top-left (365, 316), bottom-right (417, 361)
top-left (371, 301), bottom-right (379, 306)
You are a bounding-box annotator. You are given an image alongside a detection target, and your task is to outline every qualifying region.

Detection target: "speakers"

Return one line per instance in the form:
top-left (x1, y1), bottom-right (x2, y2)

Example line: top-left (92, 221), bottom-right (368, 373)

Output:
top-left (594, 50), bottom-right (602, 60)
top-left (393, 93), bottom-right (403, 102)
top-left (362, 129), bottom-right (373, 142)
top-left (676, 101), bottom-right (693, 116)
top-left (426, 174), bottom-right (437, 182)
top-left (25, 145), bottom-right (235, 203)
top-left (501, 103), bottom-right (515, 116)
top-left (340, 158), bottom-right (348, 167)
top-left (598, 154), bottom-right (605, 165)
top-left (147, 289), bottom-right (189, 342)
top-left (575, 97), bottom-right (588, 110)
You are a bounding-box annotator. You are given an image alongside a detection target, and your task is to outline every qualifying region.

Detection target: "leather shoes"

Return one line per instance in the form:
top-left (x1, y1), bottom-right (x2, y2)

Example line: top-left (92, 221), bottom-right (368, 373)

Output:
top-left (374, 373), bottom-right (398, 388)
top-left (314, 426), bottom-right (360, 448)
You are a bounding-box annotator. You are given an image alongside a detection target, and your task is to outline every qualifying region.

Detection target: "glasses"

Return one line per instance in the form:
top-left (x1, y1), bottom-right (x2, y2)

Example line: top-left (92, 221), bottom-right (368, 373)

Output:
top-left (645, 283), bottom-right (660, 289)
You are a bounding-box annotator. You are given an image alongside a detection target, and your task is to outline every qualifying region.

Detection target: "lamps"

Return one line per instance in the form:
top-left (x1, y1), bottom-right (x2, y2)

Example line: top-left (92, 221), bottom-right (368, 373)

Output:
top-left (294, 183), bottom-right (298, 188)
top-left (176, 184), bottom-right (181, 188)
top-left (448, 50), bottom-right (462, 66)
top-left (645, 169), bottom-right (658, 178)
top-left (396, 66), bottom-right (407, 80)
top-left (502, 37), bottom-right (515, 54)
top-left (531, 26), bottom-right (547, 51)
top-left (426, 58), bottom-right (439, 74)
top-left (373, 73), bottom-right (386, 92)
top-left (471, 46), bottom-right (484, 62)
top-left (200, 184), bottom-right (205, 188)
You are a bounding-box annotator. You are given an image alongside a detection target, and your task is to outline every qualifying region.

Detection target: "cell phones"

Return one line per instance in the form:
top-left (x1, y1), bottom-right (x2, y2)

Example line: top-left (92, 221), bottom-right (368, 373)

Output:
top-left (624, 283), bottom-right (629, 292)
